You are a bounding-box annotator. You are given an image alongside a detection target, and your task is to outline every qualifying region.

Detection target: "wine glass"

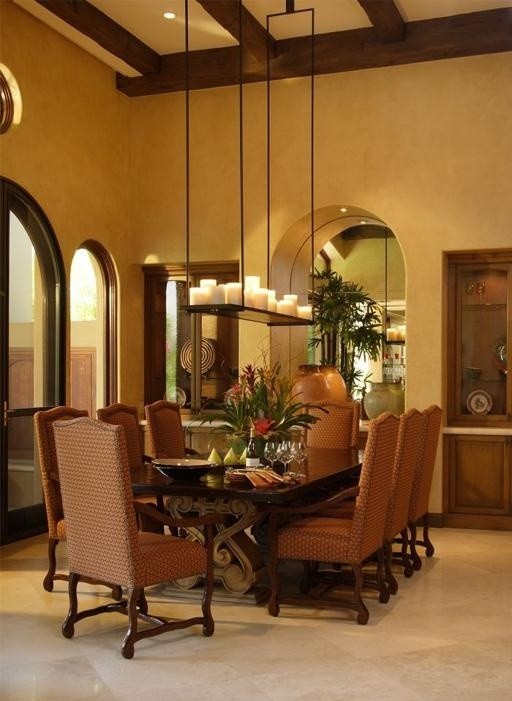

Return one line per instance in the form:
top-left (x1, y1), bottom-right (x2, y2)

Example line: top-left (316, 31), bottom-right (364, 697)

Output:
top-left (465, 282), bottom-right (477, 305)
top-left (263, 441), bottom-right (307, 480)
top-left (476, 282), bottom-right (486, 305)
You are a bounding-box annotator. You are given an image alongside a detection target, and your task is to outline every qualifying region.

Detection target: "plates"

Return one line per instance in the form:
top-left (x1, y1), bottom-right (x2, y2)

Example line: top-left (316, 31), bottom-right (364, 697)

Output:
top-left (223, 388), bottom-right (235, 410)
top-left (466, 390), bottom-right (492, 417)
top-left (212, 465), bottom-right (244, 476)
top-left (490, 337), bottom-right (506, 375)
top-left (164, 387), bottom-right (188, 410)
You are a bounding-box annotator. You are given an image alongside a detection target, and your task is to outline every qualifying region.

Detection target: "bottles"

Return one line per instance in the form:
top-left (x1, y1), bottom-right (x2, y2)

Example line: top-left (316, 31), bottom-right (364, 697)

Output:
top-left (246, 428), bottom-right (261, 470)
top-left (290, 365), bottom-right (332, 408)
top-left (362, 383), bottom-right (405, 418)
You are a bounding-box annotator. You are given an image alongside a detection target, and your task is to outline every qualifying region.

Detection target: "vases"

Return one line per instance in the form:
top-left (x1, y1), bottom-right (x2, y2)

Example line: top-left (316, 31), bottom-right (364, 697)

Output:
top-left (291, 363), bottom-right (331, 415)
top-left (364, 380), bottom-right (404, 422)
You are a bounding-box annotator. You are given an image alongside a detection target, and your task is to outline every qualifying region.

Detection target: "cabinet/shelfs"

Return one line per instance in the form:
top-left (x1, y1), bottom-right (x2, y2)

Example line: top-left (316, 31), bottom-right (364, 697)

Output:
top-left (442, 248), bottom-right (512, 531)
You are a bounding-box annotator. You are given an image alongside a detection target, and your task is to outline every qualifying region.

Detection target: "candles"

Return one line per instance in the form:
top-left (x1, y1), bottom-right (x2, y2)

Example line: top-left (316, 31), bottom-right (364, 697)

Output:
top-left (189, 276), bottom-right (312, 320)
top-left (387, 326), bottom-right (405, 342)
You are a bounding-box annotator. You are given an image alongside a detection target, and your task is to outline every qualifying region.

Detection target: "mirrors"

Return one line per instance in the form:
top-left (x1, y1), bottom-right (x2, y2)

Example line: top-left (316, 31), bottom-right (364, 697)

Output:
top-left (287, 214), bottom-right (405, 419)
top-left (139, 260), bottom-right (239, 420)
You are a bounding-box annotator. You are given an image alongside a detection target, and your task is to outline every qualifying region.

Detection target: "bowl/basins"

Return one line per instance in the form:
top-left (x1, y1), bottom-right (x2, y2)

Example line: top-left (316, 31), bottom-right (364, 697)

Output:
top-left (150, 458), bottom-right (216, 483)
top-left (226, 470), bottom-right (254, 485)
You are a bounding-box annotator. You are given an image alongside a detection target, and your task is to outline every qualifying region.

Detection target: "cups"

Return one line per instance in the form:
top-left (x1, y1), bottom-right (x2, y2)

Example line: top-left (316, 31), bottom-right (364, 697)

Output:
top-left (187, 275), bottom-right (313, 321)
top-left (386, 326), bottom-right (405, 342)
top-left (463, 368), bottom-right (481, 380)
